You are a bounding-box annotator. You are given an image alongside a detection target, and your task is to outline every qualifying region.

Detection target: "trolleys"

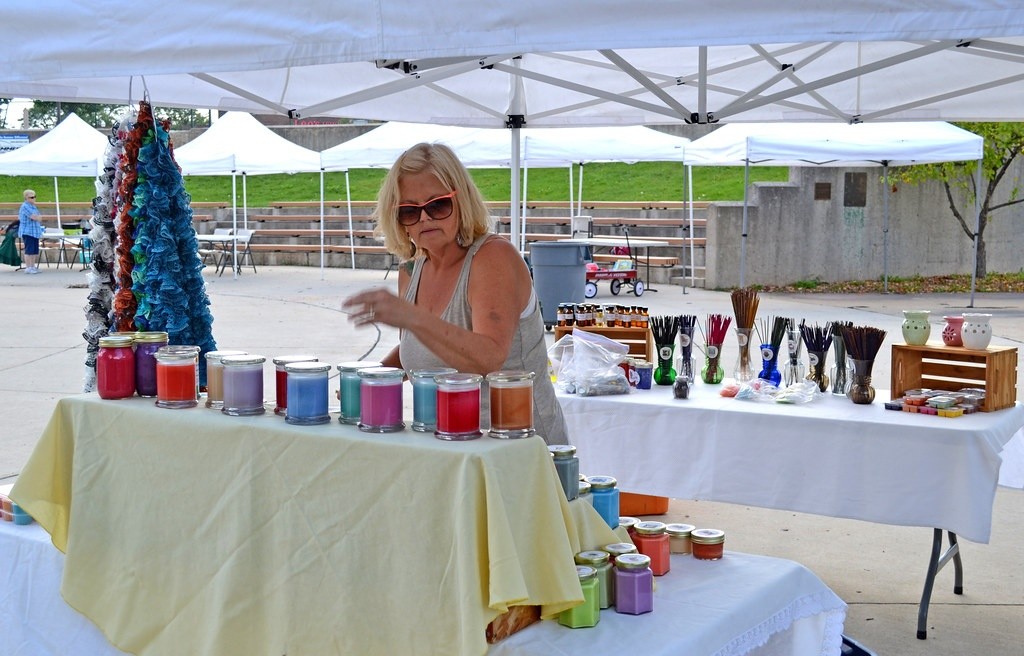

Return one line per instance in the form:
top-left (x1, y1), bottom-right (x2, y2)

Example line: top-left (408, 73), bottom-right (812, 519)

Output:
top-left (585, 227), bottom-right (644, 298)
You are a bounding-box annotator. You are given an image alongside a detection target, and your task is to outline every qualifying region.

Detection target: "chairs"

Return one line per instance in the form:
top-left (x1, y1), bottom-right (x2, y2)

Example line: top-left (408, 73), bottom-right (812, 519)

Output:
top-left (37, 228), bottom-right (70, 269)
top-left (219, 230), bottom-right (257, 277)
top-left (202, 228), bottom-right (234, 273)
top-left (70, 227), bottom-right (93, 270)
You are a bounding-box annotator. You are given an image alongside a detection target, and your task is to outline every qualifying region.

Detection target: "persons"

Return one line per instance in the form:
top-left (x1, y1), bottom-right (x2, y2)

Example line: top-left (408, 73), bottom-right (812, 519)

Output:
top-left (340, 144), bottom-right (573, 445)
top-left (17, 190), bottom-right (44, 273)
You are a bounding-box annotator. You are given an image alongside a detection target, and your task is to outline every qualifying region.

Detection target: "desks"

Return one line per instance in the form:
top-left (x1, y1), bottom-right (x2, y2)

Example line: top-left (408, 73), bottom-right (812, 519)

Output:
top-left (375, 237), bottom-right (417, 280)
top-left (552, 378), bottom-right (1024, 641)
top-left (0, 473), bottom-right (849, 656)
top-left (558, 238), bottom-right (669, 294)
top-left (196, 234), bottom-right (250, 276)
top-left (13, 233), bottom-right (91, 272)
top-left (8, 390), bottom-right (656, 656)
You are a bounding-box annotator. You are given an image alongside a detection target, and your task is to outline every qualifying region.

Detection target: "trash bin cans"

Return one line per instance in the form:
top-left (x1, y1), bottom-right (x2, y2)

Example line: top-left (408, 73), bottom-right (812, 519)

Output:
top-left (527, 240), bottom-right (593, 333)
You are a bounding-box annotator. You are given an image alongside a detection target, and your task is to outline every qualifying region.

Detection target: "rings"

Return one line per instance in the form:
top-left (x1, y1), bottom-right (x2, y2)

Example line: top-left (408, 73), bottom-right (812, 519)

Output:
top-left (370, 305), bottom-right (373, 312)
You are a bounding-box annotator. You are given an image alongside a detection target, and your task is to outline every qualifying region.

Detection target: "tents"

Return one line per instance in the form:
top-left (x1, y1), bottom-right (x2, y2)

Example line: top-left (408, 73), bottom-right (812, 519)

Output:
top-left (320, 125), bottom-right (693, 288)
top-left (682, 119), bottom-right (983, 308)
top-left (174, 110), bottom-right (355, 280)
top-left (0, 111), bottom-right (110, 264)
top-left (0, 0), bottom-right (1024, 250)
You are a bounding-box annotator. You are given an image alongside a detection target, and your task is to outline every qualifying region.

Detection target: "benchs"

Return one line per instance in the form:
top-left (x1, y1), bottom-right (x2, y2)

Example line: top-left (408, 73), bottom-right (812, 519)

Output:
top-left (0, 201), bottom-right (711, 285)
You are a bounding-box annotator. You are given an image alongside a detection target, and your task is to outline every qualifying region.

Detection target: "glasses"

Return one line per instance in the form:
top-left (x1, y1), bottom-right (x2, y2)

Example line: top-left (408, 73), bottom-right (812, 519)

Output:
top-left (27, 196), bottom-right (36, 199)
top-left (393, 189), bottom-right (458, 226)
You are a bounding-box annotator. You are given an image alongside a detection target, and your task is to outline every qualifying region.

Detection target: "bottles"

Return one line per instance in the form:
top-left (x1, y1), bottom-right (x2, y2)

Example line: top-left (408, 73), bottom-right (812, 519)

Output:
top-left (604, 543), bottom-right (638, 583)
top-left (665, 523), bottom-right (696, 555)
top-left (575, 550), bottom-right (615, 609)
top-left (556, 302), bottom-right (875, 404)
top-left (545, 444), bottom-right (580, 502)
top-left (577, 474), bottom-right (620, 530)
top-left (555, 566), bottom-right (600, 629)
top-left (95, 330), bottom-right (535, 442)
top-left (618, 517), bottom-right (671, 576)
top-left (690, 529), bottom-right (725, 560)
top-left (611, 553), bottom-right (654, 615)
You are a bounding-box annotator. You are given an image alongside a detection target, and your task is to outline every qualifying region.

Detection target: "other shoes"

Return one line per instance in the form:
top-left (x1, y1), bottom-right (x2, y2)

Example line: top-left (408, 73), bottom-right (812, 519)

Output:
top-left (31, 266), bottom-right (42, 273)
top-left (25, 266), bottom-right (37, 274)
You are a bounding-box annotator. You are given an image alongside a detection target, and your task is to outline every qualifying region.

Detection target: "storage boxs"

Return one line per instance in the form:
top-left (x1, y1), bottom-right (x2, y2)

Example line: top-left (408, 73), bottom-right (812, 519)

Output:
top-left (890, 342), bottom-right (1018, 413)
top-left (554, 324), bottom-right (653, 364)
